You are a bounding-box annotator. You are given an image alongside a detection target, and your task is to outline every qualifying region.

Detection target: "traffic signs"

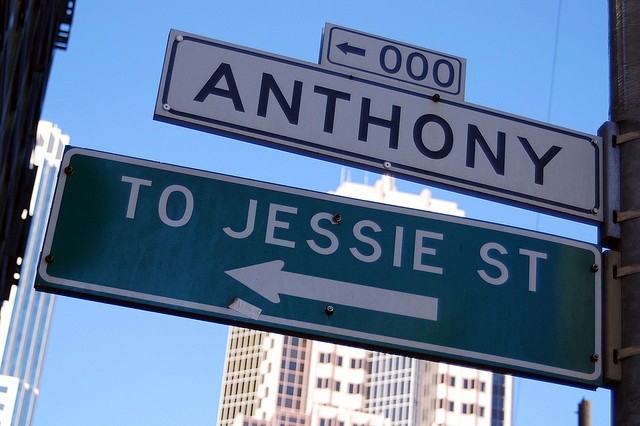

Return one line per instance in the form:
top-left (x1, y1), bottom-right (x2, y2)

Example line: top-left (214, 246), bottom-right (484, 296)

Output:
top-left (35, 144), bottom-right (604, 391)
top-left (153, 19), bottom-right (606, 224)
